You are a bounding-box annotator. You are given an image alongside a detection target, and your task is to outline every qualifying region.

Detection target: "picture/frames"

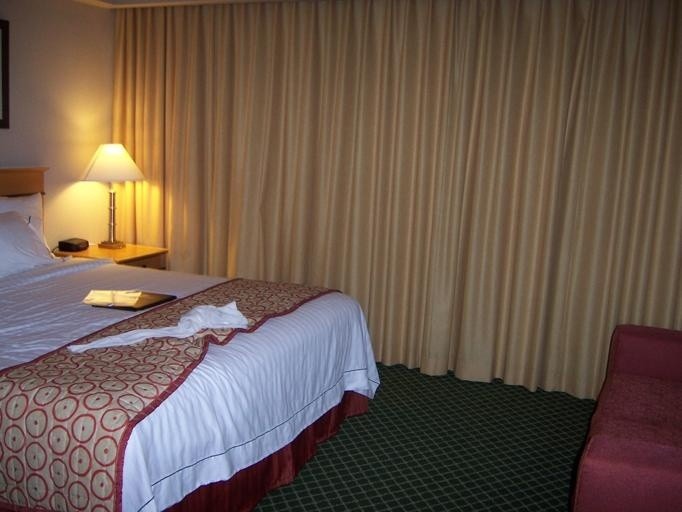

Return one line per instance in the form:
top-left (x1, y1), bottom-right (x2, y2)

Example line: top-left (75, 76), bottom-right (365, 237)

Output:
top-left (0, 18), bottom-right (10, 130)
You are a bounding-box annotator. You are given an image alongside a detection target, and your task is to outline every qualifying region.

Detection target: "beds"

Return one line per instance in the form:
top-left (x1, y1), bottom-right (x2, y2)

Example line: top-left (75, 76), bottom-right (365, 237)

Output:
top-left (0, 166), bottom-right (381, 512)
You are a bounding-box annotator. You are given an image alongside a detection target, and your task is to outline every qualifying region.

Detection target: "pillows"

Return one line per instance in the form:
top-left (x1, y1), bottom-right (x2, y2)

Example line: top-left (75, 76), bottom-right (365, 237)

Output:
top-left (0, 193), bottom-right (43, 238)
top-left (0, 211), bottom-right (66, 278)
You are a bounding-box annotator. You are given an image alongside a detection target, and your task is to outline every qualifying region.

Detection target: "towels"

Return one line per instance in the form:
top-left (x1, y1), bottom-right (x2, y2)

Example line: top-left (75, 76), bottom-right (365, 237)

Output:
top-left (66, 301), bottom-right (255, 355)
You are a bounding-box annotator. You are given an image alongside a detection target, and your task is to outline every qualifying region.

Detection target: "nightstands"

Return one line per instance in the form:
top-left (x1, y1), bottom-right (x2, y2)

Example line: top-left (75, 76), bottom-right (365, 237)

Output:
top-left (51, 243), bottom-right (169, 271)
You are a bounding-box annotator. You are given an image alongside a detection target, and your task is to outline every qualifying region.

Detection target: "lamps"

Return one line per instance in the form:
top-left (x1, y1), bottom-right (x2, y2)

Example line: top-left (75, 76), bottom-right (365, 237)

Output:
top-left (79, 142), bottom-right (146, 250)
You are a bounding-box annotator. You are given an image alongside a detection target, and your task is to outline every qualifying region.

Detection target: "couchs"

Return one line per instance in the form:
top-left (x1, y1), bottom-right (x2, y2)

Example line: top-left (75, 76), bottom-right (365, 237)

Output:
top-left (570, 323), bottom-right (682, 512)
